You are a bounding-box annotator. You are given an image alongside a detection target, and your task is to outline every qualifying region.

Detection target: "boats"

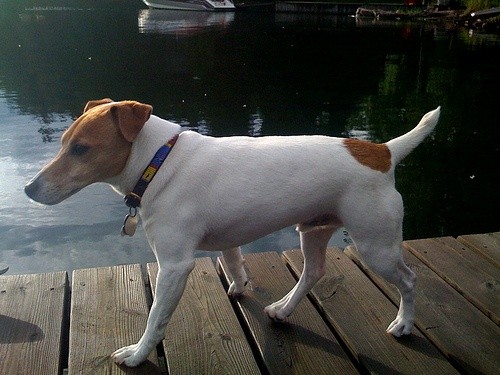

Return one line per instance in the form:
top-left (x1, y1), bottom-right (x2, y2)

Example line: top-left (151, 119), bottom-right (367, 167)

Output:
top-left (143, 0), bottom-right (236, 11)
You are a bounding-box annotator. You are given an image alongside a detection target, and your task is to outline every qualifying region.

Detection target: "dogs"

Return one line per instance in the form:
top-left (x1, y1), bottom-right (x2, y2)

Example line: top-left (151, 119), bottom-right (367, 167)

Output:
top-left (22, 97), bottom-right (442, 369)
